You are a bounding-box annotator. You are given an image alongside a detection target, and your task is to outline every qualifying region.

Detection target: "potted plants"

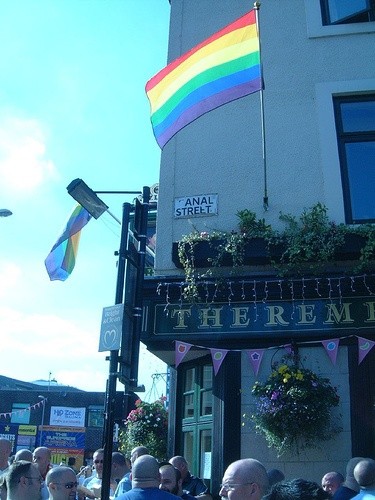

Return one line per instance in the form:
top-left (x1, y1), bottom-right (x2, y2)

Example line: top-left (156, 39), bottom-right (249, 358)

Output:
top-left (231, 201), bottom-right (375, 285)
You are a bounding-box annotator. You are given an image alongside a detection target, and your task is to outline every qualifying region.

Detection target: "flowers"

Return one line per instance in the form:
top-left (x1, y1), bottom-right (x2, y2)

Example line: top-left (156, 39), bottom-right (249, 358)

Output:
top-left (178, 218), bottom-right (227, 305)
top-left (118, 400), bottom-right (168, 461)
top-left (240, 359), bottom-right (344, 456)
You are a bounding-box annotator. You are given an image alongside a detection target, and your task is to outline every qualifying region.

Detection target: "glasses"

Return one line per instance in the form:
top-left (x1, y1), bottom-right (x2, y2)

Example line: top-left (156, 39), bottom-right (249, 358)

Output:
top-left (18, 476), bottom-right (44, 483)
top-left (93, 459), bottom-right (104, 463)
top-left (65, 481), bottom-right (79, 489)
top-left (218, 480), bottom-right (251, 491)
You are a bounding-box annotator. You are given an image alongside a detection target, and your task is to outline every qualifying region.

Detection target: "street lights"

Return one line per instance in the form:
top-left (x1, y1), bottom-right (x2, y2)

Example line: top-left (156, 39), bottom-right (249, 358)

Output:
top-left (66, 178), bottom-right (155, 258)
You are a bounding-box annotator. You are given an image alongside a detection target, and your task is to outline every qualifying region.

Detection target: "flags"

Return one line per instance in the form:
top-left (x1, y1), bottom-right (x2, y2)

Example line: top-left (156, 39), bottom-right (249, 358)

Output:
top-left (45, 204), bottom-right (92, 282)
top-left (145, 8), bottom-right (266, 150)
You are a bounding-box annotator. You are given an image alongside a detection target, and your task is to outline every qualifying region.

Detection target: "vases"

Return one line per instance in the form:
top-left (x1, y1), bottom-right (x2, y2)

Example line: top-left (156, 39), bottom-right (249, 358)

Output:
top-left (172, 240), bottom-right (231, 267)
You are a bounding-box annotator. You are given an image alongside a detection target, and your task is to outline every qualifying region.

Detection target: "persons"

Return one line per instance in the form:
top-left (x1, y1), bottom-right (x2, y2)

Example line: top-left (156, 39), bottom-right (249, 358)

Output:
top-left (14, 449), bottom-right (33, 463)
top-left (111, 452), bottom-right (132, 500)
top-left (33, 447), bottom-right (53, 480)
top-left (45, 466), bottom-right (78, 500)
top-left (76, 465), bottom-right (93, 485)
top-left (158, 464), bottom-right (181, 495)
top-left (112, 455), bottom-right (183, 500)
top-left (130, 446), bottom-right (149, 466)
top-left (5, 459), bottom-right (43, 500)
top-left (350, 460), bottom-right (375, 500)
top-left (79, 448), bottom-right (117, 500)
top-left (0, 438), bottom-right (12, 500)
top-left (67, 457), bottom-right (79, 475)
top-left (169, 455), bottom-right (213, 500)
top-left (219, 458), bottom-right (270, 500)
top-left (321, 472), bottom-right (345, 497)
top-left (332, 457), bottom-right (369, 500)
